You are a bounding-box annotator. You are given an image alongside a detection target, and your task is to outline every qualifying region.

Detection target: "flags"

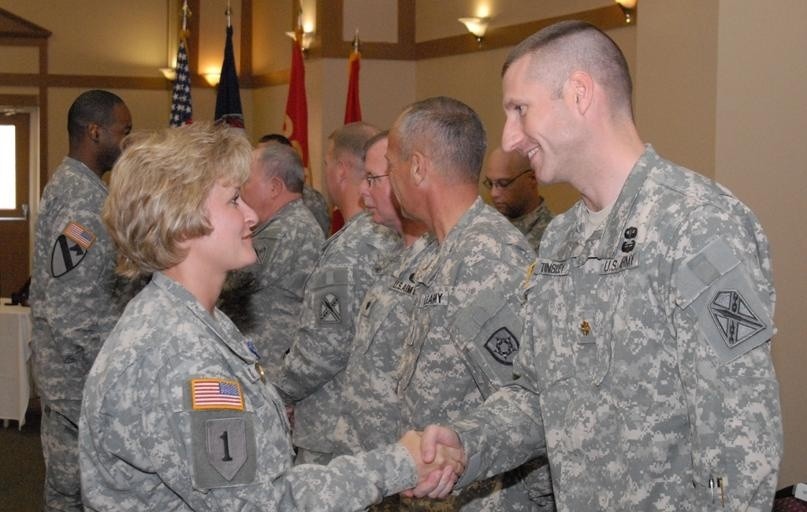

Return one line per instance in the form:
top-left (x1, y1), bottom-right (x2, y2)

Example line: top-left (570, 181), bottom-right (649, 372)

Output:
top-left (170, 4), bottom-right (197, 131)
top-left (281, 3), bottom-right (316, 193)
top-left (217, 4), bottom-right (247, 130)
top-left (345, 30), bottom-right (367, 129)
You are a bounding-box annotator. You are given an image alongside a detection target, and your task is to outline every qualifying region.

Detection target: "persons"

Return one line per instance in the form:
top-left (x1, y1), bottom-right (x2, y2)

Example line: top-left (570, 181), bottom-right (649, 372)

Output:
top-left (323, 96), bottom-right (554, 512)
top-left (267, 126), bottom-right (398, 465)
top-left (400, 19), bottom-right (784, 510)
top-left (259, 133), bottom-right (330, 238)
top-left (217, 146), bottom-right (327, 367)
top-left (329, 130), bottom-right (437, 511)
top-left (483, 146), bottom-right (553, 255)
top-left (78, 124), bottom-right (465, 511)
top-left (29, 91), bottom-right (133, 512)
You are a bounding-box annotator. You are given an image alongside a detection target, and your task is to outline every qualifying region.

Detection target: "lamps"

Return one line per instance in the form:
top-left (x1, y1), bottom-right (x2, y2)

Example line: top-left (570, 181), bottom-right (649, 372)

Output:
top-left (455, 15), bottom-right (492, 39)
top-left (285, 31), bottom-right (314, 50)
top-left (158, 67), bottom-right (177, 81)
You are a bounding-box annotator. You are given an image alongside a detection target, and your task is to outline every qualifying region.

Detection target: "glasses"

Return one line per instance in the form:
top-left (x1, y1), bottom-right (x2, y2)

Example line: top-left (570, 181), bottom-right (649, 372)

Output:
top-left (483, 168), bottom-right (534, 190)
top-left (367, 172), bottom-right (389, 187)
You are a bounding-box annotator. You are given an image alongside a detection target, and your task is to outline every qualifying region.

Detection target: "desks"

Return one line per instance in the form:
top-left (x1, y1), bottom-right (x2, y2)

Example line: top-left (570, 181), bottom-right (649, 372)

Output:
top-left (0, 298), bottom-right (33, 430)
